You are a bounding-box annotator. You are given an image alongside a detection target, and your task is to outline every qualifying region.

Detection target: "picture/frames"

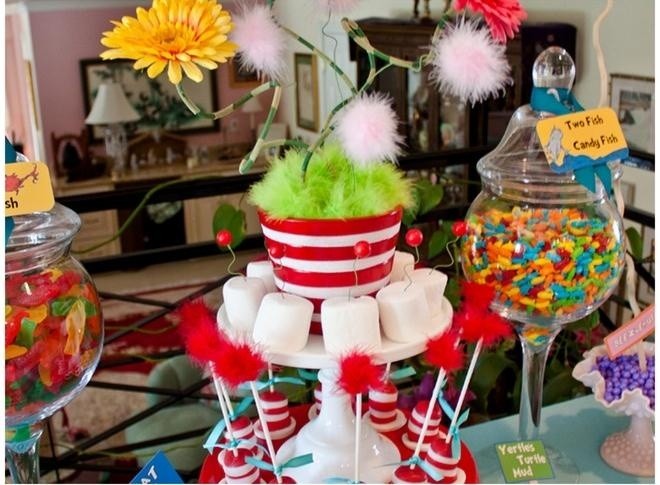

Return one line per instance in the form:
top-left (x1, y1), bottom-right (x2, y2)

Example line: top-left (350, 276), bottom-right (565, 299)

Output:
top-left (296, 52), bottom-right (319, 133)
top-left (83, 59), bottom-right (220, 144)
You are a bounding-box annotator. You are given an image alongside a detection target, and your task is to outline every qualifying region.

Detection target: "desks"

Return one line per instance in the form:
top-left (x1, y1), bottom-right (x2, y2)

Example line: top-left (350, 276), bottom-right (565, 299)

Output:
top-left (4, 205), bottom-right (655, 483)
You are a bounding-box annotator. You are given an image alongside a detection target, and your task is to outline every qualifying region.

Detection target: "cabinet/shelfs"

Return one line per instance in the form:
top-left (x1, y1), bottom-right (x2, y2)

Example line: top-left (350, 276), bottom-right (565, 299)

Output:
top-left (54, 158), bottom-right (271, 260)
top-left (357, 17), bottom-right (577, 214)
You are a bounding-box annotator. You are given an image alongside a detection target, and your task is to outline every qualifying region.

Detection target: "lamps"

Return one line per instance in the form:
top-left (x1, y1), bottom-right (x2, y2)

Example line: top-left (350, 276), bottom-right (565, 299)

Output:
top-left (86, 82), bottom-right (142, 172)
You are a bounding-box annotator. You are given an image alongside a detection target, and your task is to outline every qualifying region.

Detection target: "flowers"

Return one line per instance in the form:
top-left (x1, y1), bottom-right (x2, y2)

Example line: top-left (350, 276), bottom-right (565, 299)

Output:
top-left (99, 1), bottom-right (240, 82)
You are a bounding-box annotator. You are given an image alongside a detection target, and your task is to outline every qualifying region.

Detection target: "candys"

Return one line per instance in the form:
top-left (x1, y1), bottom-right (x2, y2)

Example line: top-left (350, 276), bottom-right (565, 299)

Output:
top-left (591, 352), bottom-right (655, 408)
top-left (461, 205), bottom-right (626, 316)
top-left (5, 262), bottom-right (104, 415)
